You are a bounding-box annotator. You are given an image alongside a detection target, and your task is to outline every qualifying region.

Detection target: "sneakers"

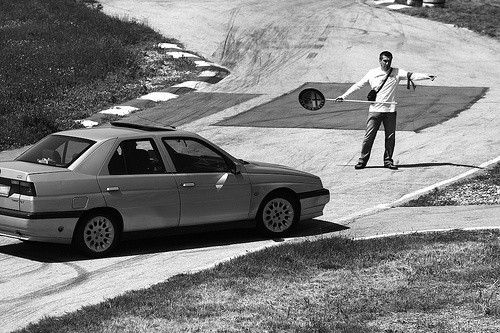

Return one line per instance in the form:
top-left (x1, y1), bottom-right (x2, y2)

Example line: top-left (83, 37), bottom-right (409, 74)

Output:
top-left (384, 164), bottom-right (397, 170)
top-left (355, 162), bottom-right (365, 169)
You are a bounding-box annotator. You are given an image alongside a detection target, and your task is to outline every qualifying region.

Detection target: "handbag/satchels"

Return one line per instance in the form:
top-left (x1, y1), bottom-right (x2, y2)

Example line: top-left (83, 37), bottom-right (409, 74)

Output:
top-left (367, 90), bottom-right (376, 101)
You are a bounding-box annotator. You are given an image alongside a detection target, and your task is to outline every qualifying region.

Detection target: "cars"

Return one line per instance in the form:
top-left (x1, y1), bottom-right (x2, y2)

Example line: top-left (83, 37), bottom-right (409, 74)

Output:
top-left (0, 122), bottom-right (330, 259)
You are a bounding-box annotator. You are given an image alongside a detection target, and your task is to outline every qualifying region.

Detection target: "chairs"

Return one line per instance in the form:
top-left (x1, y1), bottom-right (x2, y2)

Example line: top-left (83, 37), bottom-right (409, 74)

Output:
top-left (41, 150), bottom-right (61, 164)
top-left (128, 149), bottom-right (152, 173)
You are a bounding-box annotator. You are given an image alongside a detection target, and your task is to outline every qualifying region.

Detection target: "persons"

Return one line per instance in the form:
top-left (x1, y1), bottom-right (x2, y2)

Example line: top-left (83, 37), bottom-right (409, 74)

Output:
top-left (120, 139), bottom-right (138, 153)
top-left (334, 50), bottom-right (437, 169)
